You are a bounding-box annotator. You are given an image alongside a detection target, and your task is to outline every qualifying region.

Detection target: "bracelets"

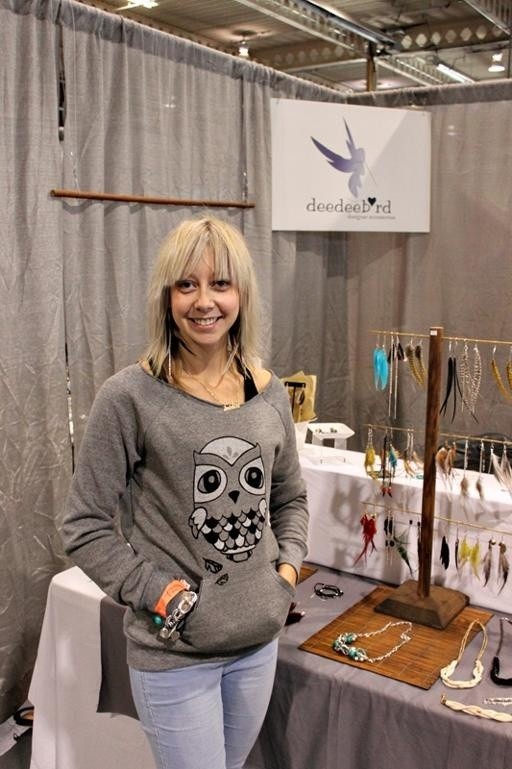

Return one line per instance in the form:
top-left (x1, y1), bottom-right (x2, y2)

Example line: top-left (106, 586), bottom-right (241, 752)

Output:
top-left (153, 579), bottom-right (190, 625)
top-left (159, 591), bottom-right (198, 640)
top-left (314, 582), bottom-right (343, 600)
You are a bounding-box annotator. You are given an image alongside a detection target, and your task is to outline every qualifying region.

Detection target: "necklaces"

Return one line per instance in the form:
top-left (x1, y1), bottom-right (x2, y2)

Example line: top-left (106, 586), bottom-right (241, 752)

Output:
top-left (489, 617), bottom-right (512, 687)
top-left (441, 695), bottom-right (512, 722)
top-left (333, 620), bottom-right (414, 663)
top-left (485, 697), bottom-right (512, 707)
top-left (182, 365), bottom-right (240, 412)
top-left (439, 620), bottom-right (488, 689)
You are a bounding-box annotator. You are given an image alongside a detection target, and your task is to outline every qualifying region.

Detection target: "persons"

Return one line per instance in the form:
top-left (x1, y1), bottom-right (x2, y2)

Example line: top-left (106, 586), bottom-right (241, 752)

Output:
top-left (60, 216), bottom-right (309, 769)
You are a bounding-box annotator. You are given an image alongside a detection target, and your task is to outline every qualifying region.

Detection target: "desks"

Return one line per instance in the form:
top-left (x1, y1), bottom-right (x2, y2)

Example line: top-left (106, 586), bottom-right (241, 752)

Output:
top-left (26, 438), bottom-right (512, 768)
